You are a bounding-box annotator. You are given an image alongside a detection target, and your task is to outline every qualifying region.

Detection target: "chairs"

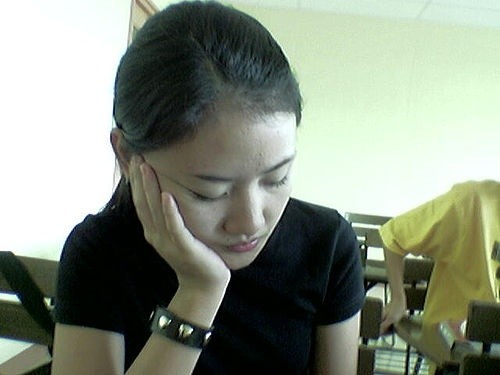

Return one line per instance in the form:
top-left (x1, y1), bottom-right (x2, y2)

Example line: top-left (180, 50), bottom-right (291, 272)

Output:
top-left (401, 258), bottom-right (440, 375)
top-left (0, 252), bottom-right (63, 375)
top-left (357, 296), bottom-right (383, 375)
top-left (463, 299), bottom-right (500, 375)
top-left (344, 211), bottom-right (399, 348)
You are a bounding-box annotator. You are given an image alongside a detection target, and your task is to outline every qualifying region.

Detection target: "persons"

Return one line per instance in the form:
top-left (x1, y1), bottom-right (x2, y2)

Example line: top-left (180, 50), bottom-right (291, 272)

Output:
top-left (378, 179), bottom-right (500, 374)
top-left (49, 1), bottom-right (365, 375)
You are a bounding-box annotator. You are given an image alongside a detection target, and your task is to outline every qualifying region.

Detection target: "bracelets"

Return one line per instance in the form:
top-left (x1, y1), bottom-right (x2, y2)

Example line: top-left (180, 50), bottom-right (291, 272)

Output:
top-left (148, 306), bottom-right (214, 349)
